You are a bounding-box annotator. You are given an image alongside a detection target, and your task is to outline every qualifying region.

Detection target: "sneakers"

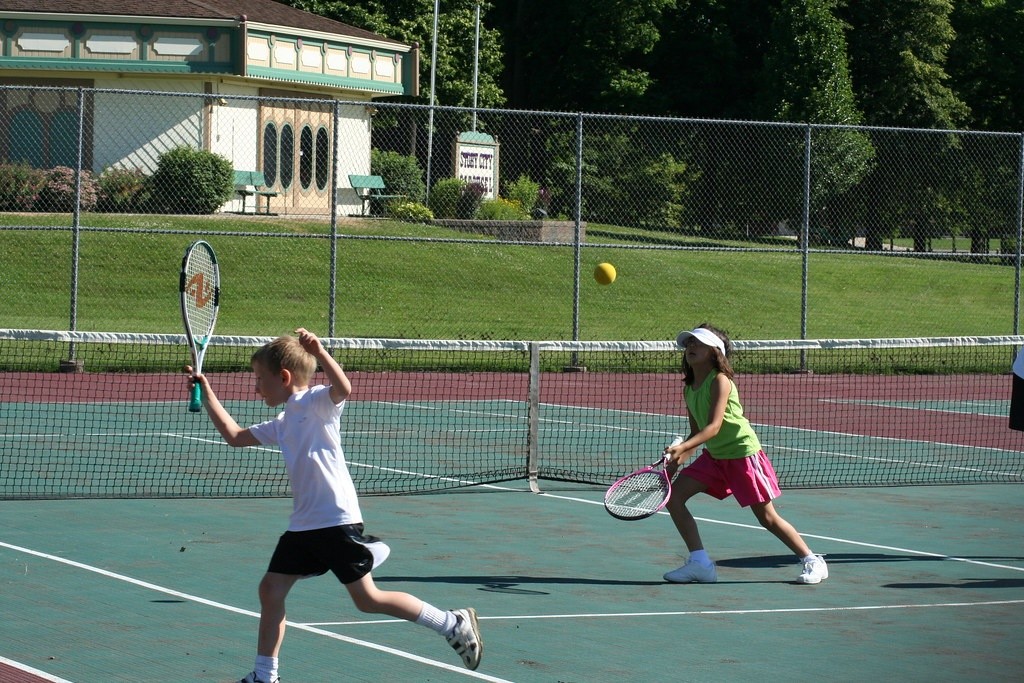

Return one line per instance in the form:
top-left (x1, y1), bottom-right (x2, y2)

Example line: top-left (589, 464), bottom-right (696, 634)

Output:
top-left (235, 671), bottom-right (281, 683)
top-left (797, 554), bottom-right (828, 584)
top-left (445, 607), bottom-right (483, 671)
top-left (662, 554), bottom-right (718, 582)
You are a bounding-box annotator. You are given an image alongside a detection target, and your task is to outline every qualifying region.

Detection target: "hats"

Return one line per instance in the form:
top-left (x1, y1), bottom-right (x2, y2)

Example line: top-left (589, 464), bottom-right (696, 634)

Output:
top-left (676, 328), bottom-right (726, 355)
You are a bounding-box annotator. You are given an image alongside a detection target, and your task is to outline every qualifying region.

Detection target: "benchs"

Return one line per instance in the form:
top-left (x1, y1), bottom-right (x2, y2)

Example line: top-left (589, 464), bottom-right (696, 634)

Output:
top-left (347, 174), bottom-right (406, 218)
top-left (227, 170), bottom-right (281, 218)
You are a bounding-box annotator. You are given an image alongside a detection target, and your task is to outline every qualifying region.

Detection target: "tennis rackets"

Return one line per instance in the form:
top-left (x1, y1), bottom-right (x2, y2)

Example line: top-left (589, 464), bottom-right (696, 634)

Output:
top-left (603, 434), bottom-right (684, 522)
top-left (179, 240), bottom-right (221, 412)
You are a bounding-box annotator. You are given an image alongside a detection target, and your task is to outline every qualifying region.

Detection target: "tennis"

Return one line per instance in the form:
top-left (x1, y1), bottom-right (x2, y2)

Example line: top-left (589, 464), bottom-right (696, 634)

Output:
top-left (593, 262), bottom-right (618, 285)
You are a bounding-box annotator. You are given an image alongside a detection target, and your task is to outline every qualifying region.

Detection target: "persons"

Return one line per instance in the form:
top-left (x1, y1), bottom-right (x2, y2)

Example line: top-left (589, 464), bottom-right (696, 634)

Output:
top-left (186, 323), bottom-right (484, 683)
top-left (663, 324), bottom-right (828, 584)
